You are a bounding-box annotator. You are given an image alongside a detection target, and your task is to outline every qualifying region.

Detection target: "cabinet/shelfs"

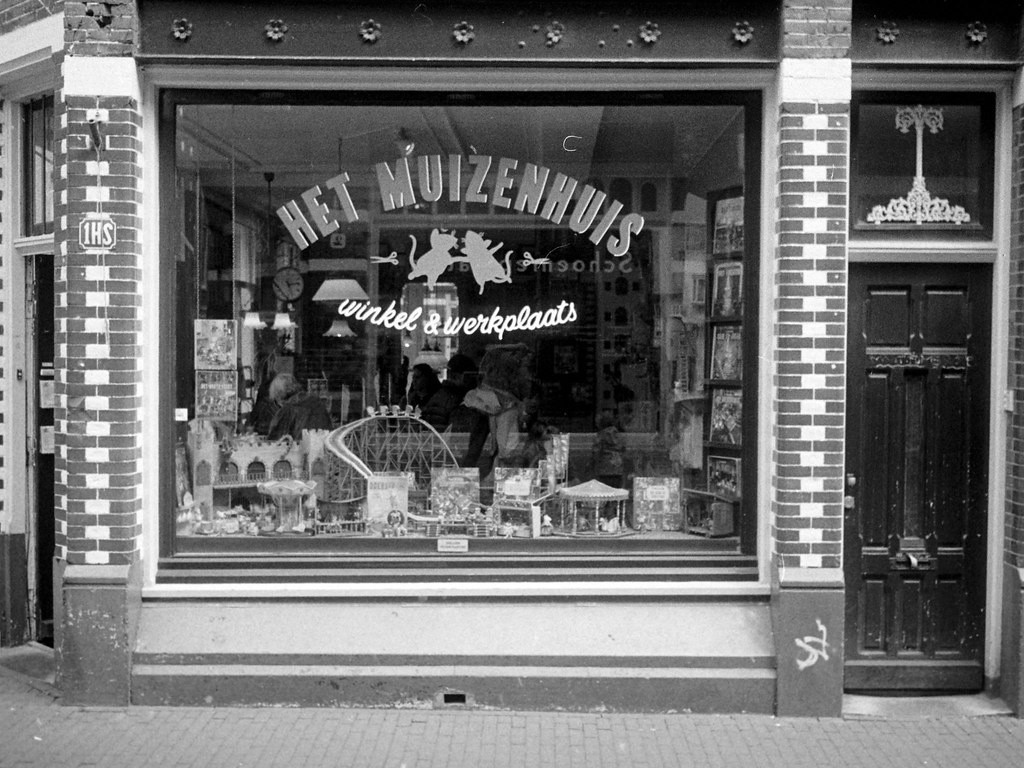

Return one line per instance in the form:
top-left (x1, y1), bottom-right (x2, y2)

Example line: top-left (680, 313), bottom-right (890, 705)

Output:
top-left (702, 183), bottom-right (745, 511)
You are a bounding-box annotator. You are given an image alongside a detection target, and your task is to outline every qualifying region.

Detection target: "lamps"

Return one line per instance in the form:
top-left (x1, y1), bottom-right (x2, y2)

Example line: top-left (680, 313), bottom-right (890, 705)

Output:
top-left (242, 302), bottom-right (296, 330)
top-left (312, 137), bottom-right (369, 301)
top-left (322, 311), bottom-right (358, 338)
top-left (393, 137), bottom-right (415, 157)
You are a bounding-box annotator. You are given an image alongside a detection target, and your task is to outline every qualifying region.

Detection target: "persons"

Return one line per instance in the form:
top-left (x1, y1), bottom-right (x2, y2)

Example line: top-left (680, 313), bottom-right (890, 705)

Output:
top-left (454, 343), bottom-right (576, 486)
top-left (400, 353), bottom-right (478, 433)
top-left (585, 411), bottom-right (624, 489)
top-left (264, 374), bottom-right (332, 442)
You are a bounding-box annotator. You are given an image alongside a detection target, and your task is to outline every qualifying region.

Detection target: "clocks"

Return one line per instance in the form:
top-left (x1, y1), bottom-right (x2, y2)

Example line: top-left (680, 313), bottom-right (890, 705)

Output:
top-left (272, 266), bottom-right (305, 303)
